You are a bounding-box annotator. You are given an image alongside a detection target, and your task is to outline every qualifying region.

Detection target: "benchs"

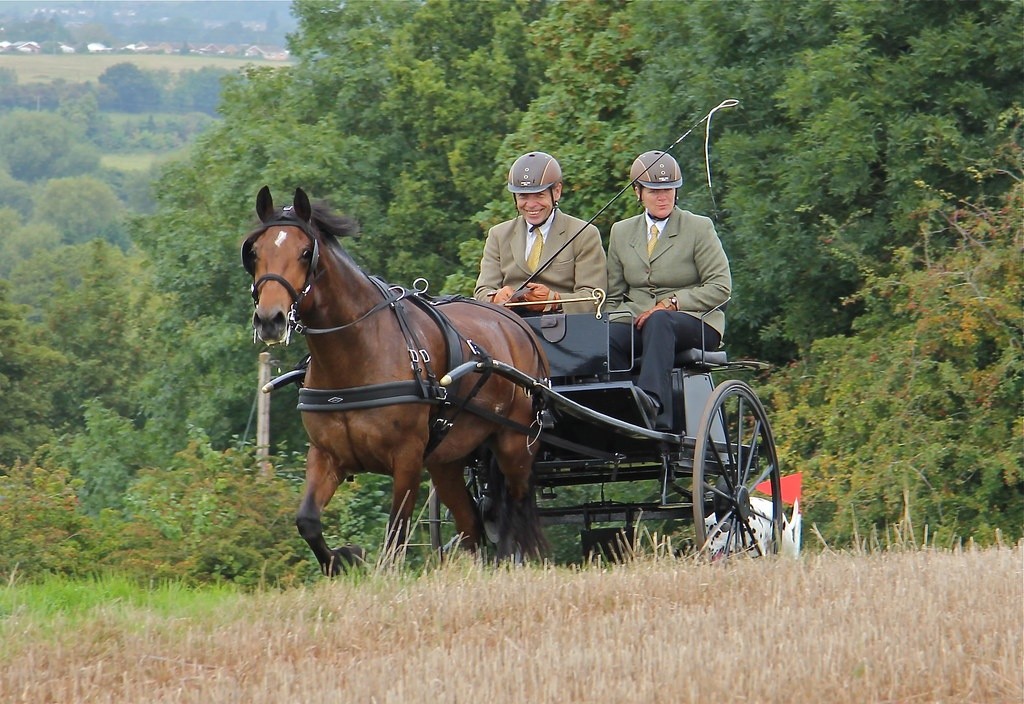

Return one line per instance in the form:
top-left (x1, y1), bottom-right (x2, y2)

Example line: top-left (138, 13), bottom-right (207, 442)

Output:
top-left (627, 347), bottom-right (727, 376)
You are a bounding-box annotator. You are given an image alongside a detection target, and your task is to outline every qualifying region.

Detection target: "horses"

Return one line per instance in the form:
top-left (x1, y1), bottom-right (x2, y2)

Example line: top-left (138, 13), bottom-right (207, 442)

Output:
top-left (247, 184), bottom-right (544, 579)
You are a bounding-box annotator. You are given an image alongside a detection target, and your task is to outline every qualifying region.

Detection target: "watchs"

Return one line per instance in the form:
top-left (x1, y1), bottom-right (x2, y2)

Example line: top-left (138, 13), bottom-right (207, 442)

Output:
top-left (670, 297), bottom-right (678, 311)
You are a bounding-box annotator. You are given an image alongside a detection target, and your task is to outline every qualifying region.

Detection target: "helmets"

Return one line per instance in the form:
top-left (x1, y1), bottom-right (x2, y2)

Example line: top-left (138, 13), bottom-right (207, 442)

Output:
top-left (629, 150), bottom-right (683, 189)
top-left (507, 151), bottom-right (563, 194)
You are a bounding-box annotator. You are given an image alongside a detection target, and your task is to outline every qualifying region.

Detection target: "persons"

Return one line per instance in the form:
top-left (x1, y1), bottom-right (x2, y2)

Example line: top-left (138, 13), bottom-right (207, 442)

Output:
top-left (474, 152), bottom-right (608, 314)
top-left (606, 149), bottom-right (732, 433)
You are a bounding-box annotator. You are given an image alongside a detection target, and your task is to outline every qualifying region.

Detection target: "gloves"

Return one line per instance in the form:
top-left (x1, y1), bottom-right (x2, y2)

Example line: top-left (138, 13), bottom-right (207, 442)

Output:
top-left (634, 299), bottom-right (677, 330)
top-left (518, 282), bottom-right (561, 312)
top-left (486, 286), bottom-right (526, 309)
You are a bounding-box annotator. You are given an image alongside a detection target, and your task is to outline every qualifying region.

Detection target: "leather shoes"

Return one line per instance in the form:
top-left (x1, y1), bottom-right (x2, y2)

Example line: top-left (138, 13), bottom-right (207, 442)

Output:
top-left (633, 385), bottom-right (661, 430)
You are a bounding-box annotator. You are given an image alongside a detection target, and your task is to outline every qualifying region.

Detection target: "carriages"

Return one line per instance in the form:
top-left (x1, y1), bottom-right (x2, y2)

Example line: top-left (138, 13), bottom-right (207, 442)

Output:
top-left (241, 184), bottom-right (784, 580)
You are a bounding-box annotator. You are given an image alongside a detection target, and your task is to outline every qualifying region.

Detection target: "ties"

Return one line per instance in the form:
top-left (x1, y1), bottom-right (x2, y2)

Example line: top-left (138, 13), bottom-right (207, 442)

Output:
top-left (648, 225), bottom-right (659, 259)
top-left (527, 228), bottom-right (543, 273)
